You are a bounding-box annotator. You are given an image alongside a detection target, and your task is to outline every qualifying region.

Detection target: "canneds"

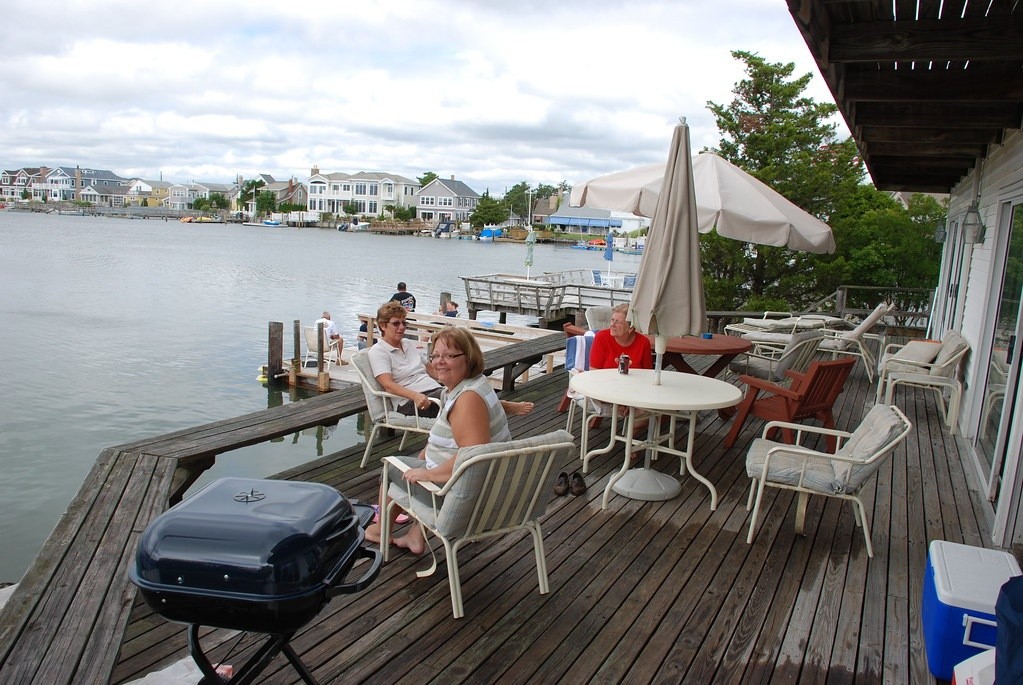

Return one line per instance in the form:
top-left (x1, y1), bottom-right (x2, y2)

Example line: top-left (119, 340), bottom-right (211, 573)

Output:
top-left (619, 355), bottom-right (629, 374)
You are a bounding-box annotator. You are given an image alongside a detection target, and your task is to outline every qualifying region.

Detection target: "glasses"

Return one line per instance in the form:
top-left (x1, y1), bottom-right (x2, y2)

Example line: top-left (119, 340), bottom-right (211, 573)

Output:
top-left (429, 351), bottom-right (465, 361)
top-left (382, 322), bottom-right (408, 327)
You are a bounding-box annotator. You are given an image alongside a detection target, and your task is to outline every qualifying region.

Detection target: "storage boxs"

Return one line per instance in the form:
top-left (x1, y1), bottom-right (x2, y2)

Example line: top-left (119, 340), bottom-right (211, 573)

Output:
top-left (920, 538), bottom-right (1023, 682)
top-left (300, 355), bottom-right (317, 367)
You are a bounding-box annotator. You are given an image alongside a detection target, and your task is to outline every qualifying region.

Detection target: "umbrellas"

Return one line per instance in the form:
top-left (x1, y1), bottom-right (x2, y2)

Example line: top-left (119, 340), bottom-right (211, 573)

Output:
top-left (623, 123), bottom-right (710, 385)
top-left (567, 153), bottom-right (836, 256)
top-left (603, 232), bottom-right (614, 276)
top-left (522, 232), bottom-right (533, 281)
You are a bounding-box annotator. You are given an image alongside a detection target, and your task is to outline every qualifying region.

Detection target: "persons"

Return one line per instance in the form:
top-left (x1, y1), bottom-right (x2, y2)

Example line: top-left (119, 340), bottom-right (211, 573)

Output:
top-left (314, 311), bottom-right (349, 365)
top-left (415, 301), bottom-right (459, 349)
top-left (357, 320), bottom-right (379, 350)
top-left (367, 301), bottom-right (535, 418)
top-left (389, 282), bottom-right (416, 312)
top-left (339, 221), bottom-right (348, 231)
top-left (365, 326), bottom-right (512, 554)
top-left (588, 303), bottom-right (654, 460)
top-left (503, 227), bottom-right (506, 238)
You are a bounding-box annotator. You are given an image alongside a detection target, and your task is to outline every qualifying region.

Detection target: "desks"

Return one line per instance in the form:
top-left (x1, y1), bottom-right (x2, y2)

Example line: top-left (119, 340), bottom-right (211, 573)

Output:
top-left (601, 277), bottom-right (623, 288)
top-left (145, 503), bottom-right (377, 685)
top-left (570, 366), bottom-right (745, 512)
top-left (646, 334), bottom-right (752, 421)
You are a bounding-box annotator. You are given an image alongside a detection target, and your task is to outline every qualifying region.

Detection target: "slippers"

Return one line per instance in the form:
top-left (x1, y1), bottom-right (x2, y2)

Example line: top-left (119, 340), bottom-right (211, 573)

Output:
top-left (371, 503), bottom-right (408, 523)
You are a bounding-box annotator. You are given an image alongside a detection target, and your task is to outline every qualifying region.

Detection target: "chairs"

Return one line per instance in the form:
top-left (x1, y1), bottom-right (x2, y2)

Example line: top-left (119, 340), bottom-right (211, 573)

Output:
top-left (379, 429), bottom-right (577, 620)
top-left (564, 333), bottom-right (629, 437)
top-left (557, 323), bottom-right (587, 414)
top-left (590, 269), bottom-right (602, 287)
top-left (744, 400), bottom-right (915, 559)
top-left (584, 305), bottom-right (612, 333)
top-left (874, 330), bottom-right (969, 435)
top-left (350, 346), bottom-right (442, 470)
top-left (728, 330), bottom-right (825, 400)
top-left (724, 301), bottom-right (897, 384)
top-left (724, 358), bottom-right (857, 454)
top-left (618, 273), bottom-right (636, 289)
top-left (303, 325), bottom-right (343, 371)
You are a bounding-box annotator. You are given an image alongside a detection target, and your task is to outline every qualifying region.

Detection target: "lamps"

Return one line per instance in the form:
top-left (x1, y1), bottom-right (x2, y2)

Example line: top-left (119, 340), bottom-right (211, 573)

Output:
top-left (934, 221), bottom-right (947, 243)
top-left (960, 200), bottom-right (986, 244)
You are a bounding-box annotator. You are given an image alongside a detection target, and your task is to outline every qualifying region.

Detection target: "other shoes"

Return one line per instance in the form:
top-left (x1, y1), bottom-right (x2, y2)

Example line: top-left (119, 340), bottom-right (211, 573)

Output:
top-left (336, 359), bottom-right (348, 364)
top-left (571, 473), bottom-right (586, 495)
top-left (554, 472), bottom-right (569, 496)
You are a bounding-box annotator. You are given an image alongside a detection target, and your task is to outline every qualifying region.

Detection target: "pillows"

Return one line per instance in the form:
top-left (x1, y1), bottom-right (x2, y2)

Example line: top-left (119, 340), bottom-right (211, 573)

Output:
top-left (893, 340), bottom-right (944, 368)
